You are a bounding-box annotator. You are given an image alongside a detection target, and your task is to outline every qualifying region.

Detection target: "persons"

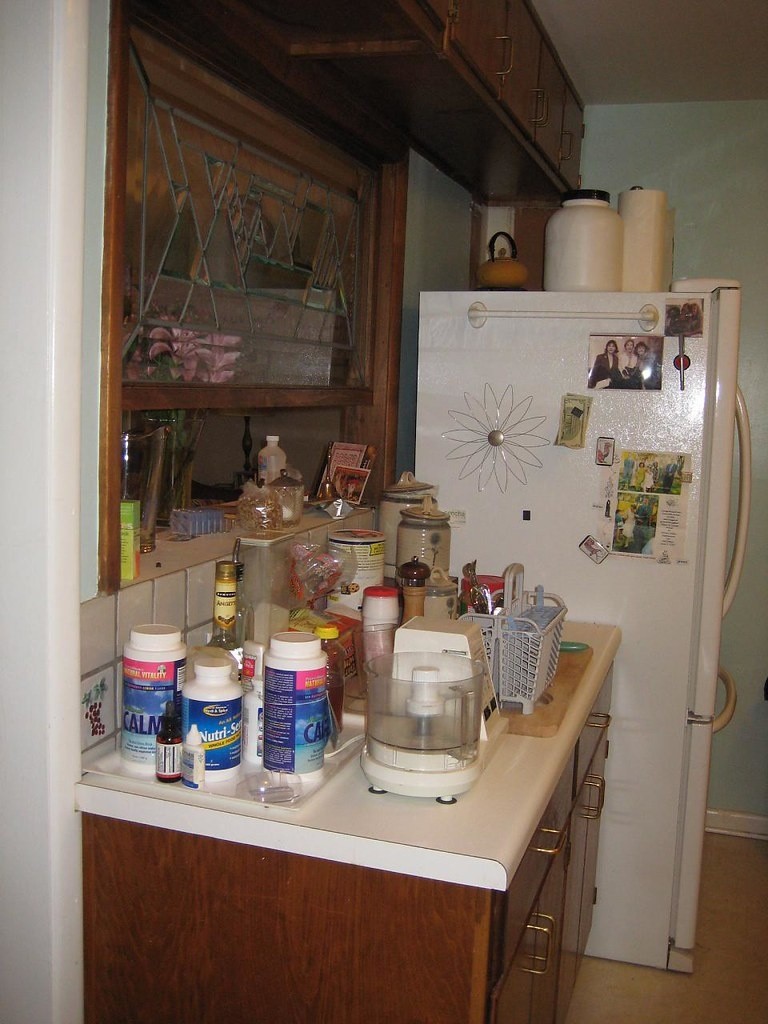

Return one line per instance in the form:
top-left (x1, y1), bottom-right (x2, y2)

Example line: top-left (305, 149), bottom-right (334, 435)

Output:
top-left (667, 303), bottom-right (702, 337)
top-left (590, 340), bottom-right (661, 390)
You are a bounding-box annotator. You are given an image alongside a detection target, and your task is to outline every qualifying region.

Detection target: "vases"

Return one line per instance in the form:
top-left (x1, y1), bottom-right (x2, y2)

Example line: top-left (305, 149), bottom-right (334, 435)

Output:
top-left (121, 421), bottom-right (174, 554)
top-left (142, 415), bottom-right (206, 529)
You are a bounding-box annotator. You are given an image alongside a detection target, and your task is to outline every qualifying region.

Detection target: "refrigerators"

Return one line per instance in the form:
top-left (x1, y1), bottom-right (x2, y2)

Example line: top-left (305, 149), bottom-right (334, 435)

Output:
top-left (415, 278), bottom-right (753, 973)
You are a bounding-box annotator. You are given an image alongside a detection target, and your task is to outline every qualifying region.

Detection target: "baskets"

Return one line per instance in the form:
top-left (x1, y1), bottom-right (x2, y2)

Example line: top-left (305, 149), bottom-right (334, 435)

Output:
top-left (456, 562), bottom-right (568, 715)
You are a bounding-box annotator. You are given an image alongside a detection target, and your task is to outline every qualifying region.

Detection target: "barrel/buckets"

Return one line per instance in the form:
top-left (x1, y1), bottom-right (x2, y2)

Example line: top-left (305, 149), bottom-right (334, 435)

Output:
top-left (327, 529), bottom-right (385, 613)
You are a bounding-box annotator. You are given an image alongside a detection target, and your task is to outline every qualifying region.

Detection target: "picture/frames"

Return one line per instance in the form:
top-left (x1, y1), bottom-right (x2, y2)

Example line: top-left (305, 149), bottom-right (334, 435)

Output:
top-left (233, 471), bottom-right (257, 490)
top-left (317, 441), bottom-right (368, 497)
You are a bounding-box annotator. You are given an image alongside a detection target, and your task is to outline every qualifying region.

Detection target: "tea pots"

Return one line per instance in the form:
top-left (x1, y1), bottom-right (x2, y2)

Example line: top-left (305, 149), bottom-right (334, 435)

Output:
top-left (477, 232), bottom-right (528, 287)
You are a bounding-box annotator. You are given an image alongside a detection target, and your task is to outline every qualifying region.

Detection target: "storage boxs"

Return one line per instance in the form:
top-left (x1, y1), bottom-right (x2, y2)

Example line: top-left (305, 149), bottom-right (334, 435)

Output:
top-left (121, 498), bottom-right (140, 530)
top-left (120, 528), bottom-right (141, 580)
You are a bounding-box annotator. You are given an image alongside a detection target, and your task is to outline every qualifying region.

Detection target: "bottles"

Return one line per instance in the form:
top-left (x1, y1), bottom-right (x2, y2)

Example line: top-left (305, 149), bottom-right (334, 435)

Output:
top-left (543, 189), bottom-right (624, 292)
top-left (399, 556), bottom-right (431, 626)
top-left (378, 470), bottom-right (451, 588)
top-left (206, 561), bottom-right (254, 686)
top-left (120, 624), bottom-right (187, 775)
top-left (182, 658), bottom-right (245, 783)
top-left (242, 680), bottom-right (264, 766)
top-left (312, 625), bottom-right (343, 735)
top-left (458, 575), bottom-right (507, 615)
top-left (257, 436), bottom-right (287, 484)
top-left (265, 632), bottom-right (327, 784)
top-left (362, 586), bottom-right (400, 672)
top-left (157, 701), bottom-right (205, 790)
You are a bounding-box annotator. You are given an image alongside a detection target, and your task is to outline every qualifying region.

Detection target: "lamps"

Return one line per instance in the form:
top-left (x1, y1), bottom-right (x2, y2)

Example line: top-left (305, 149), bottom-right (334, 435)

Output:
top-left (209, 407), bottom-right (276, 471)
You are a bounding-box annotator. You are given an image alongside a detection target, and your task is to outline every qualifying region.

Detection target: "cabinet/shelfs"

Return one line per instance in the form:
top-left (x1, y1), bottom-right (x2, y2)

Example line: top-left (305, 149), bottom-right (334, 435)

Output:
top-left (552, 661), bottom-right (615, 1024)
top-left (82, 746), bottom-right (577, 1024)
top-left (223, 0), bottom-right (587, 203)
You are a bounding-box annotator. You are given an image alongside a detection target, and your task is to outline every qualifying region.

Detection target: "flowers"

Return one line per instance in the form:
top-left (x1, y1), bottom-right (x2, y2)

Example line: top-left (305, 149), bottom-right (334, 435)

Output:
top-left (123, 302), bottom-right (240, 419)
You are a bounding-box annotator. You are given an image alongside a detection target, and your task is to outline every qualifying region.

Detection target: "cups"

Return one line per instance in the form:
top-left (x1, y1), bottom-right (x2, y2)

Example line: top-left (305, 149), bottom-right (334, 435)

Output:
top-left (424, 566), bottom-right (458, 621)
top-left (353, 623), bottom-right (398, 695)
top-left (122, 418), bottom-right (164, 553)
top-left (269, 469), bottom-right (305, 529)
